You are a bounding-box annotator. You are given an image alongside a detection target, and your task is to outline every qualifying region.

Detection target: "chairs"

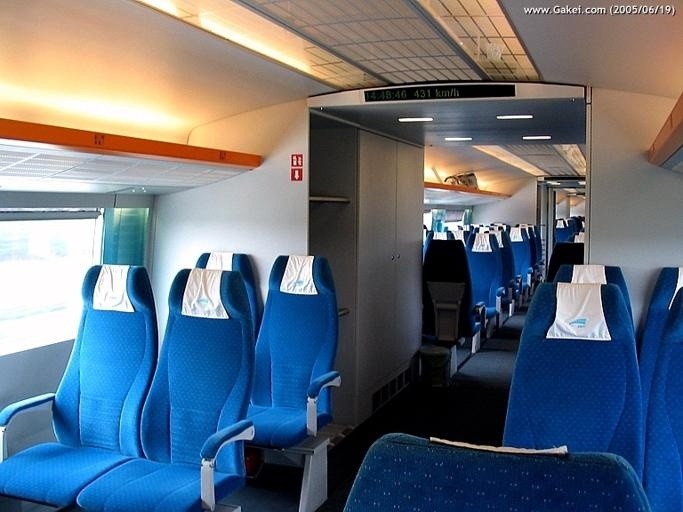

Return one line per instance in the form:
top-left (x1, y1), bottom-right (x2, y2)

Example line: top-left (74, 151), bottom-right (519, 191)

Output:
top-left (1, 215), bottom-right (682, 510)
